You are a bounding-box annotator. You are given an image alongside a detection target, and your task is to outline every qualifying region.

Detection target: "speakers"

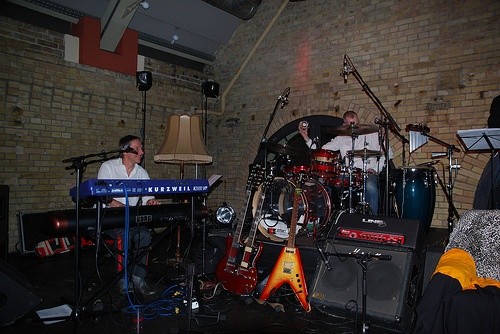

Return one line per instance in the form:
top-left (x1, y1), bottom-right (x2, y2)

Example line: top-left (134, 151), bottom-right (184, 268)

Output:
top-left (306, 238), bottom-right (425, 334)
top-left (0, 262), bottom-right (46, 329)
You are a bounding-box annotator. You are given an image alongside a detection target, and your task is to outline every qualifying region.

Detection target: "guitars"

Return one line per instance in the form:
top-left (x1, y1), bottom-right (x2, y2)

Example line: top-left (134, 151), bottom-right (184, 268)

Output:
top-left (257, 169), bottom-right (311, 314)
top-left (216, 164), bottom-right (273, 296)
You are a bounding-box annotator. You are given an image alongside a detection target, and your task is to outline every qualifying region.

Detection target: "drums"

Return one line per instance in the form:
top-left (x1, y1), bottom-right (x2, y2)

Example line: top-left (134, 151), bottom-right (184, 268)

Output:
top-left (334, 165), bottom-right (363, 189)
top-left (281, 163), bottom-right (311, 179)
top-left (251, 175), bottom-right (333, 243)
top-left (310, 147), bottom-right (339, 178)
top-left (395, 165), bottom-right (436, 235)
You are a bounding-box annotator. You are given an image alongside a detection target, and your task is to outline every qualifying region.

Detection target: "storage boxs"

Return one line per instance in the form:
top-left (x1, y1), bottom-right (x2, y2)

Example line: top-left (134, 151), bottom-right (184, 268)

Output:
top-left (17, 210), bottom-right (71, 255)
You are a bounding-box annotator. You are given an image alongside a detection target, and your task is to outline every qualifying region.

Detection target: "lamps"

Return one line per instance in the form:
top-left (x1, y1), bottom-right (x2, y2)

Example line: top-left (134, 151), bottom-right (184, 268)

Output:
top-left (140, 1), bottom-right (150, 9)
top-left (154, 113), bottom-right (213, 262)
top-left (171, 34), bottom-right (179, 44)
top-left (215, 201), bottom-right (236, 229)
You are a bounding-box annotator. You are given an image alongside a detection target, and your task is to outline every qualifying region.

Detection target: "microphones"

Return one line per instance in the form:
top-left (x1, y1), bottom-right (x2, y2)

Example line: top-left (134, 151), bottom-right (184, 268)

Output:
top-left (418, 160), bottom-right (440, 166)
top-left (124, 145), bottom-right (138, 155)
top-left (344, 55), bottom-right (348, 83)
top-left (281, 90), bottom-right (290, 108)
top-left (316, 245), bottom-right (333, 271)
top-left (374, 118), bottom-right (386, 128)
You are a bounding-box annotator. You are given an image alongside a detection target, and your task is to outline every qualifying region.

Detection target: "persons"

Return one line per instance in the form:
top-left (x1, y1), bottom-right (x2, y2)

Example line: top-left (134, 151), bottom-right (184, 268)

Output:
top-left (299, 111), bottom-right (393, 218)
top-left (93, 134), bottom-right (161, 307)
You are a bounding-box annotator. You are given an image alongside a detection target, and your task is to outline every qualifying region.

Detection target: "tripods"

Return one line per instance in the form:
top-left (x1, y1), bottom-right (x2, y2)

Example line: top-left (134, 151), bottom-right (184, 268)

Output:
top-left (343, 155), bottom-right (375, 216)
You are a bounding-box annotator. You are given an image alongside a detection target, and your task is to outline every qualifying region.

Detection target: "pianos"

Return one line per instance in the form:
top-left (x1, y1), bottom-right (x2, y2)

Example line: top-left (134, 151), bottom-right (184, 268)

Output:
top-left (69, 178), bottom-right (210, 282)
top-left (36, 203), bottom-right (212, 290)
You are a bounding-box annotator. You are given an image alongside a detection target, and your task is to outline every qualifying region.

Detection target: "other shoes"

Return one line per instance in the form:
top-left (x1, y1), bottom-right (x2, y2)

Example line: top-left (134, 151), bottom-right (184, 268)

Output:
top-left (113, 276), bottom-right (134, 294)
top-left (131, 274), bottom-right (155, 295)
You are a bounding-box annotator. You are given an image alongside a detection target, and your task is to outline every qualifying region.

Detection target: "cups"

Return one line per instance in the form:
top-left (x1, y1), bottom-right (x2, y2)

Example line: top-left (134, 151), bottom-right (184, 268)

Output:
top-left (132, 308), bottom-right (144, 331)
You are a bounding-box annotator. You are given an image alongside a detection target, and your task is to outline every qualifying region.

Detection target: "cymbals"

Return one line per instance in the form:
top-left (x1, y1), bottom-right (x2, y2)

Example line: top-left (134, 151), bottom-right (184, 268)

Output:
top-left (259, 141), bottom-right (304, 157)
top-left (332, 123), bottom-right (379, 136)
top-left (346, 149), bottom-right (383, 158)
